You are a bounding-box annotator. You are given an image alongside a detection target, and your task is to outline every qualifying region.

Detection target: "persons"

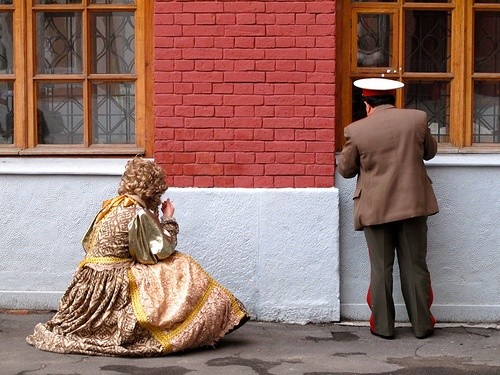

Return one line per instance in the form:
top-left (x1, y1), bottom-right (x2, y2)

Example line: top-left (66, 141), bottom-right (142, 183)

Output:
top-left (26, 154), bottom-right (252, 356)
top-left (337, 78), bottom-right (442, 339)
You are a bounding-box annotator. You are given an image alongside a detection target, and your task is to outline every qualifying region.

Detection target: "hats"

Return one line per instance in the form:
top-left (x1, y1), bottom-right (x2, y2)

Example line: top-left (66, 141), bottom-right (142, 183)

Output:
top-left (353, 78), bottom-right (404, 97)
